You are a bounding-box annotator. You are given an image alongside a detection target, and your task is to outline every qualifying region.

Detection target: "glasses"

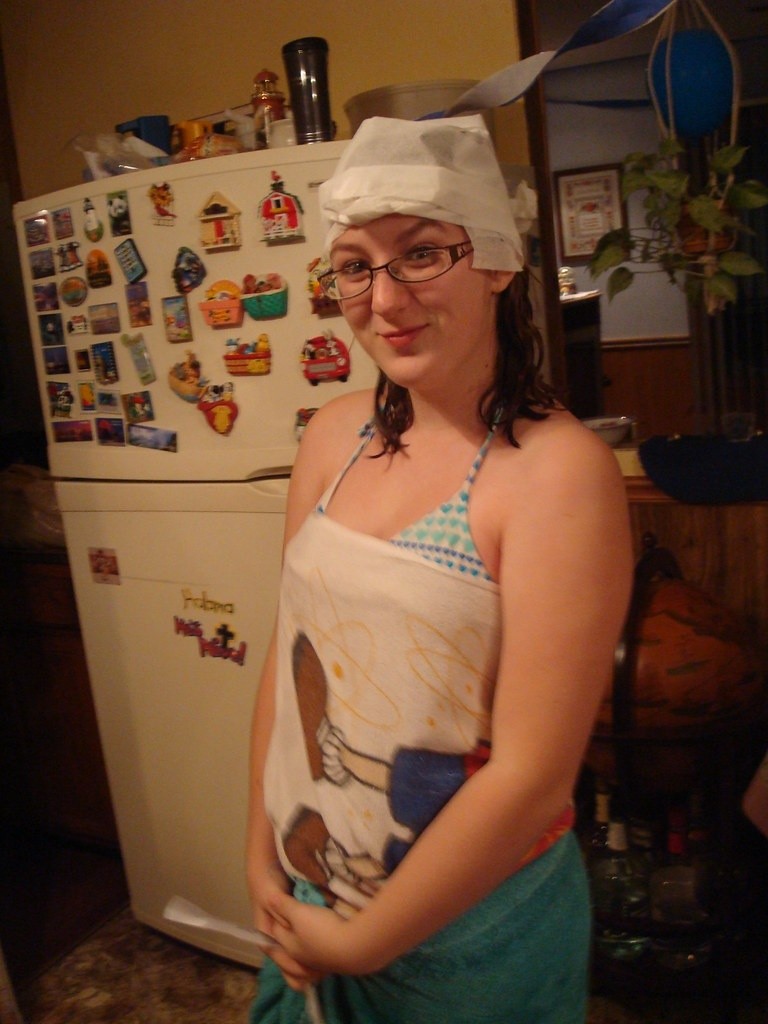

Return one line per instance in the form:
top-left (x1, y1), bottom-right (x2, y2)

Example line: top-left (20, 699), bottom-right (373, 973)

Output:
top-left (317, 239), bottom-right (472, 301)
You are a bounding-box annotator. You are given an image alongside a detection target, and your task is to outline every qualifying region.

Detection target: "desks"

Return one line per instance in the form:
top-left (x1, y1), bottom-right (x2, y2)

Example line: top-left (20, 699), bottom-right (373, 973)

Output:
top-left (562, 293), bottom-right (605, 419)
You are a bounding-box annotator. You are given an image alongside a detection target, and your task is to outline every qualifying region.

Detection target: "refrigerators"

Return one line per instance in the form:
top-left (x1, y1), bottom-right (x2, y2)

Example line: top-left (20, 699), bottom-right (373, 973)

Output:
top-left (10, 141), bottom-right (354, 971)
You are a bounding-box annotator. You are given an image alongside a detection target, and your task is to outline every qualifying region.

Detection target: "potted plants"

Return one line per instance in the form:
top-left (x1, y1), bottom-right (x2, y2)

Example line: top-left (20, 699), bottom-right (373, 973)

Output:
top-left (584, 142), bottom-right (767, 315)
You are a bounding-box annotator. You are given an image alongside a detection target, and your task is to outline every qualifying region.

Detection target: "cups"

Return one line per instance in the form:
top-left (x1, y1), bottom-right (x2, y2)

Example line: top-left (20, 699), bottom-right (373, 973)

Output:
top-left (281, 36), bottom-right (331, 145)
top-left (722, 412), bottom-right (756, 442)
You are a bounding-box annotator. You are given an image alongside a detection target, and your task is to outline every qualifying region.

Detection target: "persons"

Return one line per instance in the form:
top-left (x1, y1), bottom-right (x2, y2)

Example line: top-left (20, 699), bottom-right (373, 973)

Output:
top-left (244, 115), bottom-right (632, 1023)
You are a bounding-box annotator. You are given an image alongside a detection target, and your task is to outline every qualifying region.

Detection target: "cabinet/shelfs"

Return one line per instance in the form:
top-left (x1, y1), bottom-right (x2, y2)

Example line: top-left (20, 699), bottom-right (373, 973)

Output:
top-left (0, 561), bottom-right (121, 862)
top-left (576, 707), bottom-right (768, 1024)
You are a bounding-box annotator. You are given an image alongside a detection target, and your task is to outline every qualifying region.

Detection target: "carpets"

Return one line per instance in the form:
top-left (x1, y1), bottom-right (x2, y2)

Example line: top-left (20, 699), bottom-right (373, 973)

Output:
top-left (18, 897), bottom-right (642, 1024)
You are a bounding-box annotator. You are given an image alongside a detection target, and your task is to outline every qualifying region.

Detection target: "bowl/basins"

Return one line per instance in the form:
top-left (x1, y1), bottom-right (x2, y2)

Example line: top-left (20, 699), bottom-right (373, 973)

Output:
top-left (343, 78), bottom-right (481, 141)
top-left (583, 417), bottom-right (632, 449)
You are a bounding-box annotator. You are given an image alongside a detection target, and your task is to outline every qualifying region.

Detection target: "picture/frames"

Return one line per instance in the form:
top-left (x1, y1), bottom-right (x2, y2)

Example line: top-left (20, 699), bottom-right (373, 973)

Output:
top-left (553, 162), bottom-right (631, 263)
